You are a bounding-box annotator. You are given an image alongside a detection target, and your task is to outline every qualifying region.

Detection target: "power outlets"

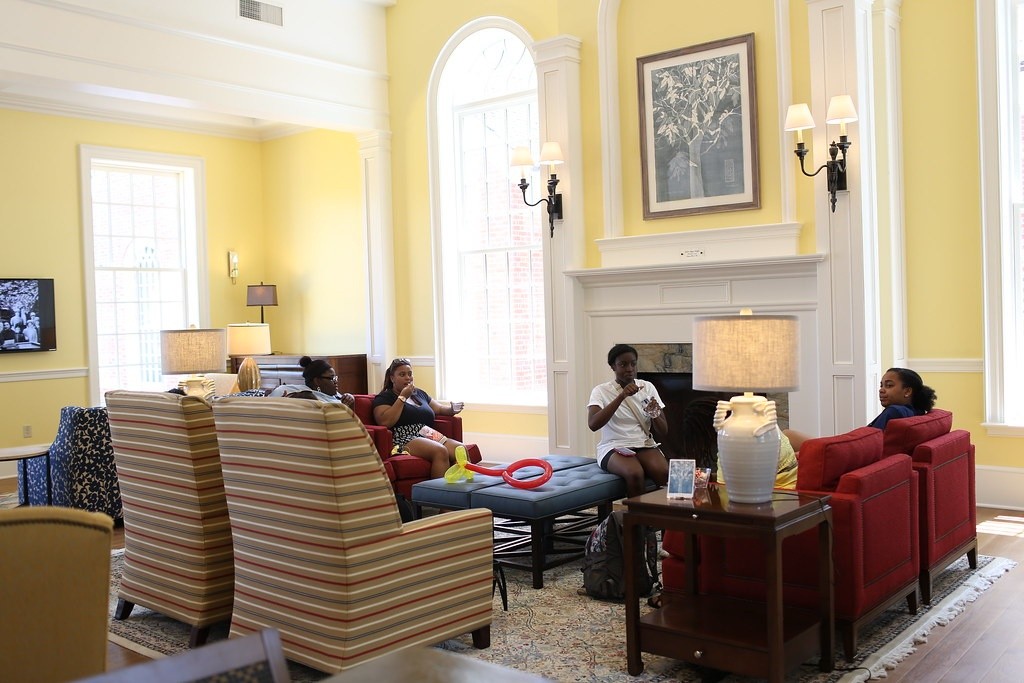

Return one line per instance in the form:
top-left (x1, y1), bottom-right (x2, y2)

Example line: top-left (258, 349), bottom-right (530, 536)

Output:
top-left (23, 425), bottom-right (33, 438)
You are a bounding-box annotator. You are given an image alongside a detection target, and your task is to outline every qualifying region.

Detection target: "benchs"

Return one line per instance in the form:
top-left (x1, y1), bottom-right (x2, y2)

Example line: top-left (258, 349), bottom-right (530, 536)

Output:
top-left (473, 454), bottom-right (659, 589)
top-left (410, 454), bottom-right (599, 520)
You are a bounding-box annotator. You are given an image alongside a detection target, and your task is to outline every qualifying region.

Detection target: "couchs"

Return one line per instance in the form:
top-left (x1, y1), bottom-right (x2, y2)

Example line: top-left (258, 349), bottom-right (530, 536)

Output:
top-left (212, 395), bottom-right (493, 677)
top-left (356, 394), bottom-right (482, 517)
top-left (911, 430), bottom-right (979, 603)
top-left (0, 506), bottom-right (113, 683)
top-left (17, 406), bottom-right (124, 521)
top-left (661, 454), bottom-right (920, 662)
top-left (105, 389), bottom-right (236, 649)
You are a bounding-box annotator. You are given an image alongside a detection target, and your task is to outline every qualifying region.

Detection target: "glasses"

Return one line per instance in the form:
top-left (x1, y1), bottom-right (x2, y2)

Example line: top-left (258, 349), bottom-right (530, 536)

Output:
top-left (319, 375), bottom-right (339, 382)
top-left (391, 358), bottom-right (411, 372)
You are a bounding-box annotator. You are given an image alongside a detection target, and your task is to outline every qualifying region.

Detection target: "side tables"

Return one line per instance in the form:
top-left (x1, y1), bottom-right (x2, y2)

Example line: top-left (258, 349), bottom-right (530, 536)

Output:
top-left (0, 446), bottom-right (52, 507)
top-left (622, 479), bottom-right (838, 683)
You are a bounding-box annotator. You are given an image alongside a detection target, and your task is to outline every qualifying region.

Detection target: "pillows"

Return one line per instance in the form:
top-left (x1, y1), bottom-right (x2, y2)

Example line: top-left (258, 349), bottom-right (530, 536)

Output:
top-left (883, 408), bottom-right (952, 455)
top-left (797, 426), bottom-right (884, 491)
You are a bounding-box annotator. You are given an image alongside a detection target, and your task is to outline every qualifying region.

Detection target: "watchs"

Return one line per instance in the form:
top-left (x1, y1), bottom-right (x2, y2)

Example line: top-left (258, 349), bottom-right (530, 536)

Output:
top-left (398, 396), bottom-right (407, 402)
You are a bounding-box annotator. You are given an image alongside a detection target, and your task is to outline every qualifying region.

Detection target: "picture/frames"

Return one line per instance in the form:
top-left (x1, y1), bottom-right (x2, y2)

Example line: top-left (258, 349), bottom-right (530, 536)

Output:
top-left (635, 30), bottom-right (766, 222)
top-left (667, 459), bottom-right (696, 499)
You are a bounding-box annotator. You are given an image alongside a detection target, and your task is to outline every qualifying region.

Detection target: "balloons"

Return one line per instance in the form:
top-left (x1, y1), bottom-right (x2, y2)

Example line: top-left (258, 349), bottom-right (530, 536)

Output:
top-left (445, 444), bottom-right (553, 488)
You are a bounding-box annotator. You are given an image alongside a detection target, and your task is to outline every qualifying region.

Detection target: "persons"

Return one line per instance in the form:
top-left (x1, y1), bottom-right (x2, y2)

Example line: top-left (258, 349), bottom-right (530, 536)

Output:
top-left (781, 368), bottom-right (937, 463)
top-left (587, 345), bottom-right (668, 511)
top-left (0, 309), bottom-right (39, 346)
top-left (268, 356), bottom-right (355, 407)
top-left (647, 421), bottom-right (798, 610)
top-left (372, 357), bottom-right (471, 514)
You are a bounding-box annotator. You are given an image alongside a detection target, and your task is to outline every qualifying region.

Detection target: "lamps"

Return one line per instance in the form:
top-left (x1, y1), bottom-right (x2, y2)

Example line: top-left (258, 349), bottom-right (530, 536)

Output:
top-left (693, 308), bottom-right (802, 504)
top-left (227, 249), bottom-right (239, 285)
top-left (161, 324), bottom-right (227, 397)
top-left (784, 95), bottom-right (860, 214)
top-left (509, 142), bottom-right (565, 240)
top-left (247, 281), bottom-right (280, 324)
top-left (227, 320), bottom-right (273, 393)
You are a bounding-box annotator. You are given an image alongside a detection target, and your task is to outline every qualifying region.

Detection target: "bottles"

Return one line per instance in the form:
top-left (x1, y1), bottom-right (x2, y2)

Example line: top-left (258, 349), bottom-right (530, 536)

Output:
top-left (637, 388), bottom-right (661, 418)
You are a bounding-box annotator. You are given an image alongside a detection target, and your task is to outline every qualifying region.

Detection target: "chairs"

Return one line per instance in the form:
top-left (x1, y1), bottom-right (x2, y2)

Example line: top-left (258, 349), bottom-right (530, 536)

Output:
top-left (78, 625), bottom-right (292, 683)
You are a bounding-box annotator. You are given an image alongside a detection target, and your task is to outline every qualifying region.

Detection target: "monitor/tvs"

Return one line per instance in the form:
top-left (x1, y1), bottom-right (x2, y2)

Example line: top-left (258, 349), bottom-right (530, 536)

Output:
top-left (0, 278), bottom-right (57, 354)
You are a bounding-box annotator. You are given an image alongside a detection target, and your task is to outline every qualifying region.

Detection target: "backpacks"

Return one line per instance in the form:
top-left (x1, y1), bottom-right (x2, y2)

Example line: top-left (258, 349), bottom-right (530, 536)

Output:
top-left (580, 510), bottom-right (663, 608)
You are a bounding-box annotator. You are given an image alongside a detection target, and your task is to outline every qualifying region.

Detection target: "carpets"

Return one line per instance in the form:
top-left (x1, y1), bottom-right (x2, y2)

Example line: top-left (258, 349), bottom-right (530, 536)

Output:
top-left (107, 546), bottom-right (1016, 683)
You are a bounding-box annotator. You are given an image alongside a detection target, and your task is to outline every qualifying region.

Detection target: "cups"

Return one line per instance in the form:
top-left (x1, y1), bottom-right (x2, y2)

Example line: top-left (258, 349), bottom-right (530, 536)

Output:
top-left (452, 399), bottom-right (462, 411)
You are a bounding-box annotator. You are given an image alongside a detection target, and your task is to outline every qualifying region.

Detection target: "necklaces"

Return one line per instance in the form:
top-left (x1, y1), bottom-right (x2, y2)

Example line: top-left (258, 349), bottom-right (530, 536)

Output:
top-left (393, 390), bottom-right (399, 395)
